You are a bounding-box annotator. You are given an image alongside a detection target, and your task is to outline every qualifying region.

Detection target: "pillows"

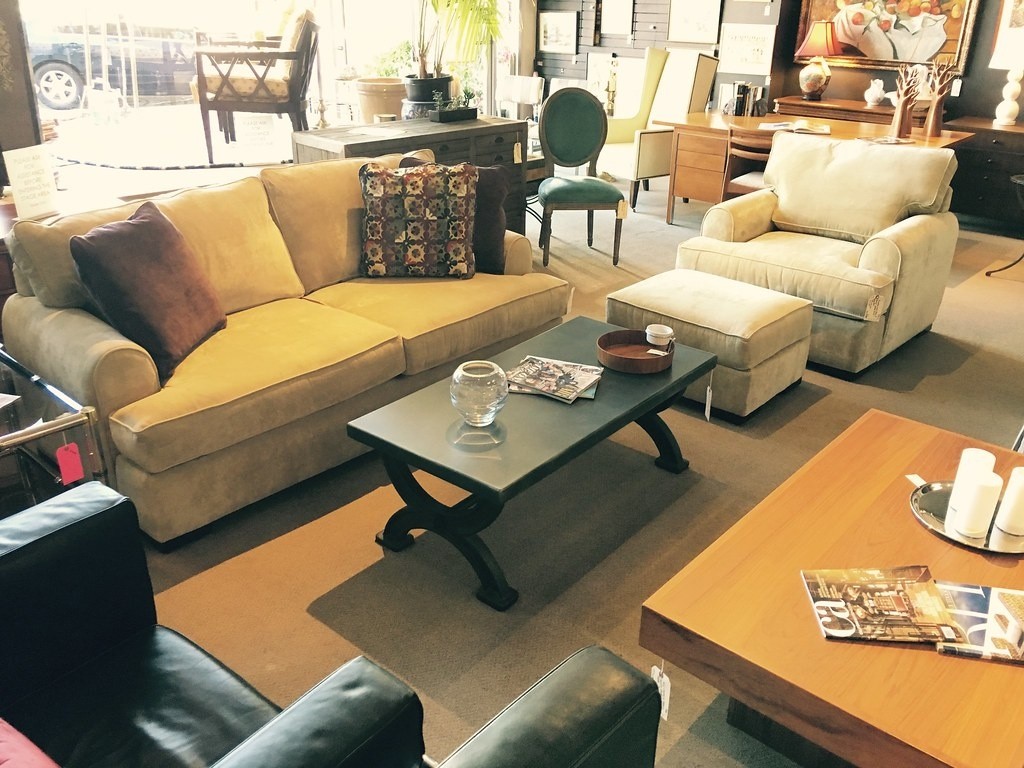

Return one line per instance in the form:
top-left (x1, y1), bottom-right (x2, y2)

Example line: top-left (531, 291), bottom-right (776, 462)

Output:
top-left (399, 156), bottom-right (509, 275)
top-left (359, 160), bottom-right (478, 281)
top-left (70, 198), bottom-right (228, 378)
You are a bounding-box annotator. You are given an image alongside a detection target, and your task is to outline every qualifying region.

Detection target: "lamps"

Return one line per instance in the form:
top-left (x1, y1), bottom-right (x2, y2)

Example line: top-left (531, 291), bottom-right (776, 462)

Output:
top-left (792, 20), bottom-right (846, 102)
top-left (988, 27), bottom-right (1024, 126)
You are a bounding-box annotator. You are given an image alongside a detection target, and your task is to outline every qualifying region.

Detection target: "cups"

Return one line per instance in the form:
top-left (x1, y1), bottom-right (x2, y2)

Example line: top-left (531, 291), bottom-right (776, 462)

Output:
top-left (644, 324), bottom-right (674, 352)
top-left (950, 448), bottom-right (1024, 538)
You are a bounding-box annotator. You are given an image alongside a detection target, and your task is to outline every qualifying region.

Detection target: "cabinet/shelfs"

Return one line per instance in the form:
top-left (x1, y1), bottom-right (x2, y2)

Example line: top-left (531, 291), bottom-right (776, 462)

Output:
top-left (291, 113), bottom-right (528, 239)
top-left (0, 347), bottom-right (111, 521)
top-left (942, 117), bottom-right (1024, 227)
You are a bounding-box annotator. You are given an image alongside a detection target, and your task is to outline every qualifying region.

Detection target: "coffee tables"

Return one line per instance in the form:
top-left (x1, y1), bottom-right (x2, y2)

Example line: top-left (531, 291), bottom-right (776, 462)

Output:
top-left (344, 315), bottom-right (717, 612)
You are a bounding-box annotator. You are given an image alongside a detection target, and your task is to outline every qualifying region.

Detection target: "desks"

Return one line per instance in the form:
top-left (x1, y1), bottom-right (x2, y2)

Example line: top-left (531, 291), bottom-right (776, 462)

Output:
top-left (651, 113), bottom-right (975, 224)
top-left (640, 408), bottom-right (1024, 768)
top-left (772, 95), bottom-right (948, 129)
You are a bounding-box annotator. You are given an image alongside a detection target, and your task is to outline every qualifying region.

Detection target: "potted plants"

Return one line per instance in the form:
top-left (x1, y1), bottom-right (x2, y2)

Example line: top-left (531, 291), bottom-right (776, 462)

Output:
top-left (428, 89), bottom-right (477, 123)
top-left (404, 0), bottom-right (500, 102)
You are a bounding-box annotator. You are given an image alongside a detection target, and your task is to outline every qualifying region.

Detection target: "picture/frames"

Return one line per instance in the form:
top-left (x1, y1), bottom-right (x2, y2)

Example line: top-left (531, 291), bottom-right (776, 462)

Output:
top-left (537, 9), bottom-right (579, 55)
top-left (793, 0), bottom-right (980, 79)
top-left (992, 0), bottom-right (1024, 54)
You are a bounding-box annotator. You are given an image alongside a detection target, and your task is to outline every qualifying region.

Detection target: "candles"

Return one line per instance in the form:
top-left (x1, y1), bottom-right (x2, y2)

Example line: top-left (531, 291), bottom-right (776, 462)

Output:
top-left (994, 466), bottom-right (1024, 536)
top-left (951, 468), bottom-right (1003, 539)
top-left (948, 448), bottom-right (996, 512)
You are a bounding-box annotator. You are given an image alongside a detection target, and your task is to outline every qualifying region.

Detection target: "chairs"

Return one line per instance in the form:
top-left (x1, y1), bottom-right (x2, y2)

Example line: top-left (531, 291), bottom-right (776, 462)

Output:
top-left (587, 47), bottom-right (721, 213)
top-left (538, 87), bottom-right (628, 268)
top-left (188, 8), bottom-right (323, 165)
top-left (494, 74), bottom-right (545, 156)
top-left (549, 78), bottom-right (600, 98)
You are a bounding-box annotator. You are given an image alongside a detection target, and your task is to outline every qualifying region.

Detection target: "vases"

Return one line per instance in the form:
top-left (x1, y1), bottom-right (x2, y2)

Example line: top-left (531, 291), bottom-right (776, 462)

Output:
top-left (401, 97), bottom-right (457, 120)
top-left (864, 79), bottom-right (886, 106)
top-left (451, 360), bottom-right (509, 428)
top-left (332, 78), bottom-right (361, 100)
top-left (351, 76), bottom-right (408, 124)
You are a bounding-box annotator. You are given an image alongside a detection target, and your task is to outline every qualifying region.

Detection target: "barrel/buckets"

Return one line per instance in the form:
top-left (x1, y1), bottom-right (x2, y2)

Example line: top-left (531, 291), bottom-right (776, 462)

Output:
top-left (356, 77), bottom-right (407, 123)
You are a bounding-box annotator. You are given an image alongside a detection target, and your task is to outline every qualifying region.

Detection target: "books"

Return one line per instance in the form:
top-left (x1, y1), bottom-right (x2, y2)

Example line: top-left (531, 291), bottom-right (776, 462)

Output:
top-left (933, 580), bottom-right (1024, 664)
top-left (733, 81), bottom-right (758, 117)
top-left (800, 566), bottom-right (964, 643)
top-left (506, 357), bottom-right (601, 404)
top-left (759, 120), bottom-right (831, 135)
top-left (509, 355), bottom-right (604, 399)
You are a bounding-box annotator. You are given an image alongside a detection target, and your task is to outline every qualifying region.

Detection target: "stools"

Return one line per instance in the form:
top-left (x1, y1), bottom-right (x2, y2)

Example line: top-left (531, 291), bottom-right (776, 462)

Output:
top-left (608, 269), bottom-right (814, 427)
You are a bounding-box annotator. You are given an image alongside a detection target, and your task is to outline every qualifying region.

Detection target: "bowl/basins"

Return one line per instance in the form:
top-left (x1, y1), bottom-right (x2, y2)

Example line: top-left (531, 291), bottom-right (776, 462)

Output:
top-left (888, 92), bottom-right (932, 111)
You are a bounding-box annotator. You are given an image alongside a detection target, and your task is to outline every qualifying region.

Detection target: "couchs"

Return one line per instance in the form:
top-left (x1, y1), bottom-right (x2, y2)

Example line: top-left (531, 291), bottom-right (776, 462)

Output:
top-left (0, 479), bottom-right (661, 768)
top-left (1, 148), bottom-right (573, 554)
top-left (682, 129), bottom-right (960, 383)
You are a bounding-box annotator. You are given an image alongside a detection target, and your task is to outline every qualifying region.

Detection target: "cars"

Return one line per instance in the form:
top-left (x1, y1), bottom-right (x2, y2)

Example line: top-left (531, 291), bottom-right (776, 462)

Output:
top-left (30, 15), bottom-right (211, 110)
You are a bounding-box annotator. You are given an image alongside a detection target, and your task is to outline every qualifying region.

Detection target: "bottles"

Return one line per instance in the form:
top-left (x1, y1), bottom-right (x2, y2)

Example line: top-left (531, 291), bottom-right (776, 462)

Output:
top-left (864, 79), bottom-right (885, 106)
top-left (449, 359), bottom-right (509, 427)
